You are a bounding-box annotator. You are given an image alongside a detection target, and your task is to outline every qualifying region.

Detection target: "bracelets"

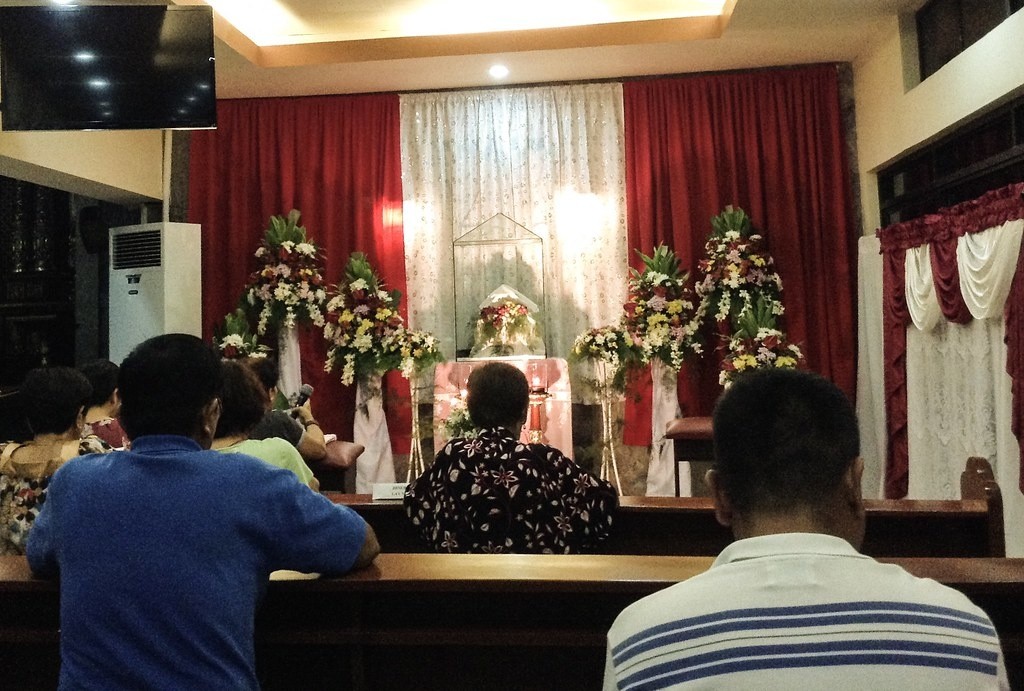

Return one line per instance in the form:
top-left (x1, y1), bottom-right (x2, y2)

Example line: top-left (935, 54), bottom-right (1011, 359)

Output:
top-left (304, 420), bottom-right (320, 430)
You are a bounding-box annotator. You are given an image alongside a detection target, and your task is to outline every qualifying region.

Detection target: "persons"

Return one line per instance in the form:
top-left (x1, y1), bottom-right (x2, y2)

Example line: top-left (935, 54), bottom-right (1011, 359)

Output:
top-left (601, 367), bottom-right (1012, 691)
top-left (242, 357), bottom-right (330, 462)
top-left (25, 333), bottom-right (381, 691)
top-left (0, 363), bottom-right (114, 556)
top-left (207, 359), bottom-right (320, 493)
top-left (403, 362), bottom-right (619, 555)
top-left (79, 358), bottom-right (129, 449)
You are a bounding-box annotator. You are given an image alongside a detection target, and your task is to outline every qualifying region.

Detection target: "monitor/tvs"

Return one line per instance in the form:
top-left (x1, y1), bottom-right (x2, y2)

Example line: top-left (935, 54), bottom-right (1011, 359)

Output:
top-left (0, 6), bottom-right (217, 131)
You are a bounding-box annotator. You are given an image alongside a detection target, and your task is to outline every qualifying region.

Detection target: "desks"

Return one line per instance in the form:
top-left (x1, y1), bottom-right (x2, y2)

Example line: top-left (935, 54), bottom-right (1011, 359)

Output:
top-left (317, 457), bottom-right (1006, 558)
top-left (0, 551), bottom-right (1024, 691)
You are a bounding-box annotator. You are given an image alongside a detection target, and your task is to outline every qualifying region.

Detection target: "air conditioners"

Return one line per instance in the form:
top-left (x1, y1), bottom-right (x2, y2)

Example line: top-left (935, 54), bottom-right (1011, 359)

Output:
top-left (108, 223), bottom-right (202, 369)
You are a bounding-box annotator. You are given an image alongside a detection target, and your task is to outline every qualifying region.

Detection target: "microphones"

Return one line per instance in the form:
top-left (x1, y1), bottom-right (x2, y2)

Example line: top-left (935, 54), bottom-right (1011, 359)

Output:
top-left (290, 384), bottom-right (313, 420)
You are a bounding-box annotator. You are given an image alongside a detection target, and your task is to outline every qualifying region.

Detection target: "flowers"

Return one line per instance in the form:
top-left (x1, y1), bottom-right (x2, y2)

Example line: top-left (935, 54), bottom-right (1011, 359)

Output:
top-left (242, 207), bottom-right (328, 356)
top-left (713, 328), bottom-right (811, 392)
top-left (321, 249), bottom-right (441, 419)
top-left (395, 329), bottom-right (445, 381)
top-left (616, 241), bottom-right (698, 378)
top-left (469, 301), bottom-right (540, 356)
top-left (698, 210), bottom-right (784, 334)
top-left (571, 325), bottom-right (626, 364)
top-left (213, 306), bottom-right (271, 369)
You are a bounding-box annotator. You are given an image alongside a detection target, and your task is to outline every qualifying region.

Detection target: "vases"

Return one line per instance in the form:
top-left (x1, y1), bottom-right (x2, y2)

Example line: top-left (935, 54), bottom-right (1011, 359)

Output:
top-left (353, 368), bottom-right (398, 495)
top-left (645, 356), bottom-right (694, 498)
top-left (272, 317), bottom-right (304, 408)
